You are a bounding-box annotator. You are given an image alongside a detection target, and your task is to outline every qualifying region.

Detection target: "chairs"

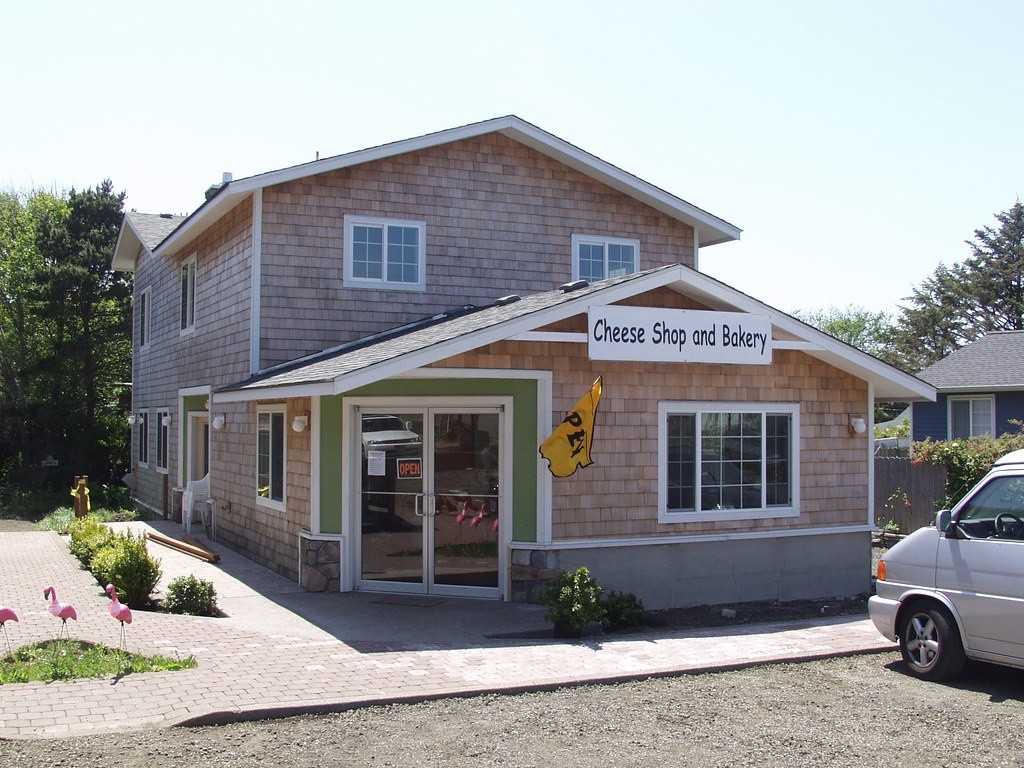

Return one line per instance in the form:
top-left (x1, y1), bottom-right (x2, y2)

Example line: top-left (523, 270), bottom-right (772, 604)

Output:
top-left (183, 471), bottom-right (209, 535)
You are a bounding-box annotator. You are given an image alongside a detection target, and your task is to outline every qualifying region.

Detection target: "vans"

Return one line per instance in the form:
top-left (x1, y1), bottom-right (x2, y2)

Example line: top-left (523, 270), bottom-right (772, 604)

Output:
top-left (867, 448), bottom-right (1024, 683)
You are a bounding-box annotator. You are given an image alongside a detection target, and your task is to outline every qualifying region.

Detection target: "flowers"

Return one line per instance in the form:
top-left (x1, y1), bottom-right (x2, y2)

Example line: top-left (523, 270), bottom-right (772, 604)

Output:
top-left (536, 565), bottom-right (609, 631)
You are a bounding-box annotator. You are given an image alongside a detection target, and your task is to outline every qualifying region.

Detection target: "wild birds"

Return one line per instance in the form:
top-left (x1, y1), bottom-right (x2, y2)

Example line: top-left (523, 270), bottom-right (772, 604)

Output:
top-left (105, 583), bottom-right (133, 653)
top-left (43, 585), bottom-right (79, 647)
top-left (455, 500), bottom-right (499, 544)
top-left (0, 607), bottom-right (19, 657)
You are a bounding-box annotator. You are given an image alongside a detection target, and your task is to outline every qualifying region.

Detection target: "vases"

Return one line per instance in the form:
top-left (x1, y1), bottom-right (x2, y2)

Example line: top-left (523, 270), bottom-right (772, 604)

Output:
top-left (552, 619), bottom-right (583, 639)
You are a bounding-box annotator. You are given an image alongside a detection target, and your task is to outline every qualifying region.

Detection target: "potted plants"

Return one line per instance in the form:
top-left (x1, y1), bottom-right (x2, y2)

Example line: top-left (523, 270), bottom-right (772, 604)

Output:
top-left (602, 589), bottom-right (645, 634)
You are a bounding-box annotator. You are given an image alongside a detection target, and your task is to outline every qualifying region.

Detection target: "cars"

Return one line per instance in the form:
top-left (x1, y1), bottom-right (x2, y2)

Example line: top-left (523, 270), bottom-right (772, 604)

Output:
top-left (361, 412), bottom-right (423, 460)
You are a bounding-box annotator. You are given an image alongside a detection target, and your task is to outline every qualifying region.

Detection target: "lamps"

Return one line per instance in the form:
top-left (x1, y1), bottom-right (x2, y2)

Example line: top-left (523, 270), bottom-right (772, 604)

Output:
top-left (292, 416), bottom-right (310, 433)
top-left (212, 416), bottom-right (226, 430)
top-left (161, 415), bottom-right (173, 428)
top-left (850, 416), bottom-right (868, 434)
top-left (128, 415), bottom-right (137, 425)
